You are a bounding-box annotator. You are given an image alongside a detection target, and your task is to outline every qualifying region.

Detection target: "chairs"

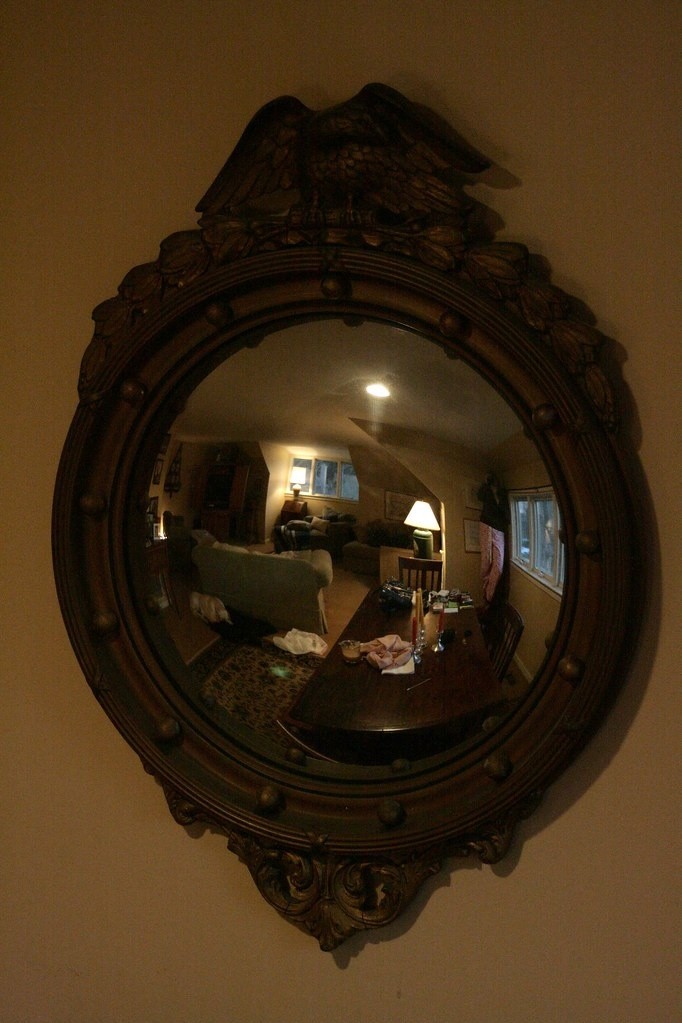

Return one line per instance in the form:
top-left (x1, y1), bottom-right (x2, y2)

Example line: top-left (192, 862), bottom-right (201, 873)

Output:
top-left (397, 555), bottom-right (443, 592)
top-left (488, 601), bottom-right (525, 684)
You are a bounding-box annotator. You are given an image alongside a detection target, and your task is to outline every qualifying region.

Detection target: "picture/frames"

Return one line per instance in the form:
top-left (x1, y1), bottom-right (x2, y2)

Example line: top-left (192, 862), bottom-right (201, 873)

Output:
top-left (153, 458), bottom-right (164, 485)
top-left (149, 496), bottom-right (159, 516)
top-left (463, 476), bottom-right (483, 511)
top-left (159, 433), bottom-right (171, 455)
top-left (384, 489), bottom-right (422, 522)
top-left (463, 516), bottom-right (481, 554)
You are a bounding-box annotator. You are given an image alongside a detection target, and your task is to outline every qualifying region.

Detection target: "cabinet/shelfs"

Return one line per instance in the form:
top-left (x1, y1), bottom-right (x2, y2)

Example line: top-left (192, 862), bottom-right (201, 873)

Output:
top-left (191, 463), bottom-right (251, 542)
top-left (281, 499), bottom-right (308, 524)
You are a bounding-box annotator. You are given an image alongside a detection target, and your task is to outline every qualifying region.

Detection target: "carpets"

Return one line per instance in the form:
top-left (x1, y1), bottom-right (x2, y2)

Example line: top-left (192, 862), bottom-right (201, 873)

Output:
top-left (186, 636), bottom-right (325, 748)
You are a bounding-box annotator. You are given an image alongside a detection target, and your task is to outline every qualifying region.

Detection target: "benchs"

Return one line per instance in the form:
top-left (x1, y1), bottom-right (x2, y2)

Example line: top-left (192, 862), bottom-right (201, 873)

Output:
top-left (342, 541), bottom-right (380, 577)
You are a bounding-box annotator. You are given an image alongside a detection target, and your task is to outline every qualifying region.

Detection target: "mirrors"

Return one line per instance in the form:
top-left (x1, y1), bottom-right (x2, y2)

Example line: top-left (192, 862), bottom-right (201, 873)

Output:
top-left (48, 81), bottom-right (654, 952)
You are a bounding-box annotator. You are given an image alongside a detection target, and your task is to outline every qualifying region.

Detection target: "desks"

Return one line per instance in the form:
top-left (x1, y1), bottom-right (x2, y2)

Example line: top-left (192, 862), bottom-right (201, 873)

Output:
top-left (145, 539), bottom-right (182, 621)
top-left (288, 587), bottom-right (508, 759)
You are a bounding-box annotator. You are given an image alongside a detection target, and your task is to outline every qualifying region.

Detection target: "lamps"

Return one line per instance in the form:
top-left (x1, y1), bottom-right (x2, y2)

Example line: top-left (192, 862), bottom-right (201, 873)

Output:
top-left (404, 499), bottom-right (441, 560)
top-left (289, 465), bottom-right (307, 501)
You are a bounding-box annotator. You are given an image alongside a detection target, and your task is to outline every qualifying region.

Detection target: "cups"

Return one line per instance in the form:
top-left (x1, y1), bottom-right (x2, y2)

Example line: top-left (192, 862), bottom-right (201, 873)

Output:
top-left (340, 640), bottom-right (362, 663)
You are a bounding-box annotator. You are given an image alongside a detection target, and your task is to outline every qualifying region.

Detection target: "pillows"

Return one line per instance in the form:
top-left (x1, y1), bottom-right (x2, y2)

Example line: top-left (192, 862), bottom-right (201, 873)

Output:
top-left (311, 516), bottom-right (329, 533)
top-left (287, 519), bottom-right (311, 531)
top-left (352, 522), bottom-right (373, 543)
top-left (322, 504), bottom-right (338, 522)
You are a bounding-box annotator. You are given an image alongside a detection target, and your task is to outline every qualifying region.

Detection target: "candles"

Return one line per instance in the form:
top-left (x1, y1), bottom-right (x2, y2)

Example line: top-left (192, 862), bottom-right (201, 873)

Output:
top-left (417, 588), bottom-right (426, 629)
top-left (415, 592), bottom-right (421, 621)
top-left (438, 609), bottom-right (445, 631)
top-left (412, 617), bottom-right (418, 645)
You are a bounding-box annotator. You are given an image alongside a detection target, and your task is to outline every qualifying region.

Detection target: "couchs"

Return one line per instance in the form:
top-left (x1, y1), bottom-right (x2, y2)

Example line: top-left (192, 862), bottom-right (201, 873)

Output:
top-left (162, 511), bottom-right (191, 541)
top-left (190, 530), bottom-right (334, 636)
top-left (304, 514), bottom-right (351, 563)
